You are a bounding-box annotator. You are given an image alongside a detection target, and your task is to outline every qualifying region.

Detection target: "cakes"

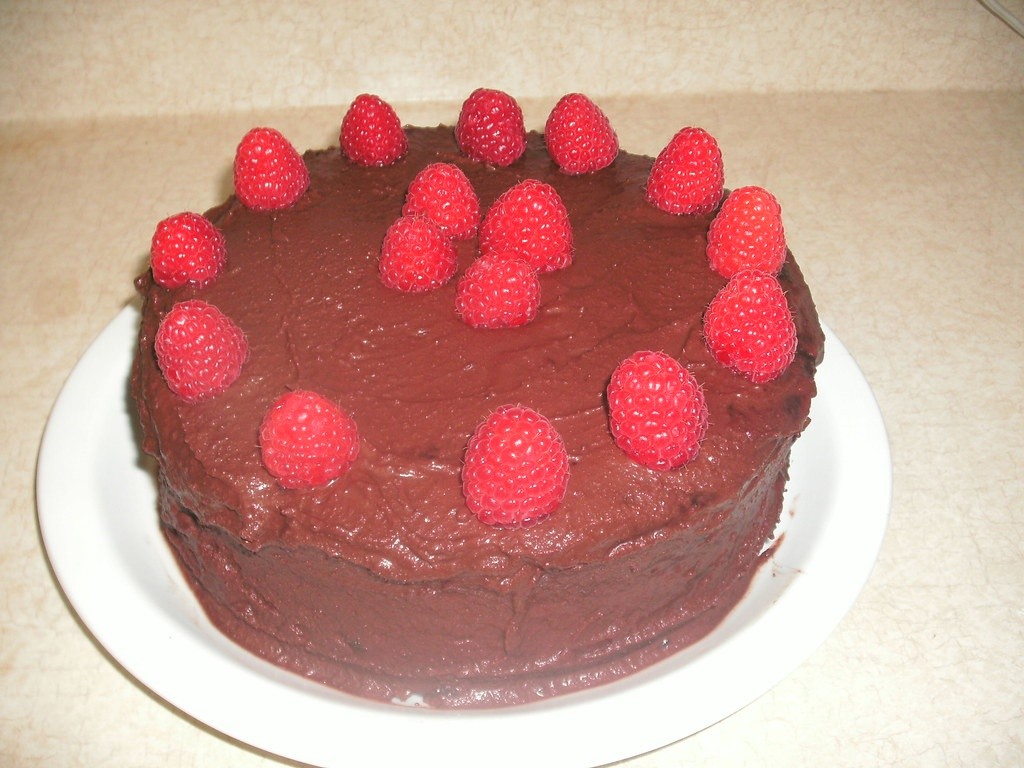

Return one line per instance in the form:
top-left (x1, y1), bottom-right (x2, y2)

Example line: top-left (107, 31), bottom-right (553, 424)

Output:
top-left (129, 86), bottom-right (826, 711)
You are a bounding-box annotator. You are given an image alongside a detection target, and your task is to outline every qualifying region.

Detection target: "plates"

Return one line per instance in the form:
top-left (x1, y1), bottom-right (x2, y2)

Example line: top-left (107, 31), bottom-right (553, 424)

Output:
top-left (35, 278), bottom-right (893, 768)
